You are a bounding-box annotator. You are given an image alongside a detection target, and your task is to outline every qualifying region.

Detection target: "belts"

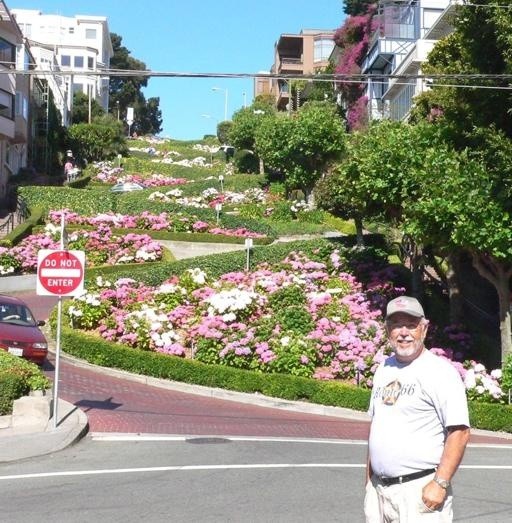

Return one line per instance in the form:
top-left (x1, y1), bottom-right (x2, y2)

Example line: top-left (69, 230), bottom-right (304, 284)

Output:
top-left (379, 468), bottom-right (435, 486)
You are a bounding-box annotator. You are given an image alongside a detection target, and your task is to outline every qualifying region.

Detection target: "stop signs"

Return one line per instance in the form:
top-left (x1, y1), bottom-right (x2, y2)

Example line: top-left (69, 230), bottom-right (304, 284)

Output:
top-left (35, 249), bottom-right (85, 296)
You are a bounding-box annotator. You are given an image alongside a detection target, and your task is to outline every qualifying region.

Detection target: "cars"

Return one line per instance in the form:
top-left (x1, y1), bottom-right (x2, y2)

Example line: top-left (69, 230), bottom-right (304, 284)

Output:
top-left (1, 293), bottom-right (48, 365)
top-left (109, 181), bottom-right (147, 193)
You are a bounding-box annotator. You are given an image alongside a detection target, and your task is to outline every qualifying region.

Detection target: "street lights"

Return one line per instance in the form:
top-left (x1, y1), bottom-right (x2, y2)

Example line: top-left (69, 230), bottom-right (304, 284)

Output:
top-left (87, 75), bottom-right (113, 124)
top-left (201, 114), bottom-right (219, 129)
top-left (212, 86), bottom-right (228, 120)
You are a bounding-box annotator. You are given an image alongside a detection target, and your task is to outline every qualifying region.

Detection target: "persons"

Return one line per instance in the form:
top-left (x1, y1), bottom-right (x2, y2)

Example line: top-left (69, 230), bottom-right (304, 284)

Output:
top-left (363, 295), bottom-right (471, 523)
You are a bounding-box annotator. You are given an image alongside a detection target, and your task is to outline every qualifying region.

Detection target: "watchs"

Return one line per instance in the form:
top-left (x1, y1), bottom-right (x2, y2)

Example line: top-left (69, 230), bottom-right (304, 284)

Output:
top-left (433, 474), bottom-right (450, 490)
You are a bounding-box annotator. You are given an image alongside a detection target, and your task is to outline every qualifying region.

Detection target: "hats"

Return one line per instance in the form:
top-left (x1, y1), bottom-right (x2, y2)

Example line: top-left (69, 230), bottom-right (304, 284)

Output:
top-left (385, 295), bottom-right (424, 321)
